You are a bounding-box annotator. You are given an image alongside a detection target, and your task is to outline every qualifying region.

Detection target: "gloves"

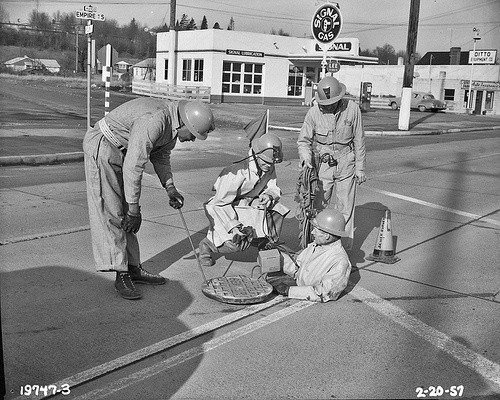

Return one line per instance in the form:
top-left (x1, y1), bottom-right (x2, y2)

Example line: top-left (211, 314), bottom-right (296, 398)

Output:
top-left (264, 242), bottom-right (277, 250)
top-left (121, 204), bottom-right (142, 234)
top-left (274, 283), bottom-right (289, 296)
top-left (165, 184), bottom-right (184, 209)
top-left (233, 226), bottom-right (253, 251)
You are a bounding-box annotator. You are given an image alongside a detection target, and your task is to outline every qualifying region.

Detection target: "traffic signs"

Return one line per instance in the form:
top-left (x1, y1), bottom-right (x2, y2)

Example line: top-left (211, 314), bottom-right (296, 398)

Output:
top-left (75, 10), bottom-right (106, 22)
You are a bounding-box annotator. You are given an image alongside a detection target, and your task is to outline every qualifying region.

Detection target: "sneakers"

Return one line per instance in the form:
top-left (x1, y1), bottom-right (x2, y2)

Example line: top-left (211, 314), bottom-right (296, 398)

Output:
top-left (128, 264), bottom-right (166, 285)
top-left (199, 242), bottom-right (214, 266)
top-left (253, 236), bottom-right (285, 245)
top-left (115, 271), bottom-right (141, 299)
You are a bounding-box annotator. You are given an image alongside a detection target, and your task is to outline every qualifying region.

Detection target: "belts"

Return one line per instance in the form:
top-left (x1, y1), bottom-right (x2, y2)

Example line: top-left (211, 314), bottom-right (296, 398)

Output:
top-left (99, 117), bottom-right (127, 154)
top-left (313, 143), bottom-right (354, 162)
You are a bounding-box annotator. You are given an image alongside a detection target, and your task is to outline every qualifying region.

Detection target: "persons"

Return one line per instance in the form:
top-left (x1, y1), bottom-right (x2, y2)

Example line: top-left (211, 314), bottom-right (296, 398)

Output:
top-left (297, 76), bottom-right (367, 250)
top-left (83, 96), bottom-right (216, 300)
top-left (268, 207), bottom-right (353, 304)
top-left (197, 134), bottom-right (291, 267)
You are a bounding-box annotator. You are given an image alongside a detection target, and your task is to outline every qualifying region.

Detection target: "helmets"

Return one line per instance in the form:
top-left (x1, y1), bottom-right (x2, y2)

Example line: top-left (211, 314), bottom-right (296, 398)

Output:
top-left (252, 134), bottom-right (283, 164)
top-left (179, 100), bottom-right (215, 141)
top-left (315, 76), bottom-right (346, 105)
top-left (309, 209), bottom-right (349, 238)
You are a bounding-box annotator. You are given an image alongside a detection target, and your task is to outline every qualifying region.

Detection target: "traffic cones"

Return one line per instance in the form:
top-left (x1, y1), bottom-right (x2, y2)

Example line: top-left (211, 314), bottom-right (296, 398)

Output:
top-left (364, 209), bottom-right (401, 265)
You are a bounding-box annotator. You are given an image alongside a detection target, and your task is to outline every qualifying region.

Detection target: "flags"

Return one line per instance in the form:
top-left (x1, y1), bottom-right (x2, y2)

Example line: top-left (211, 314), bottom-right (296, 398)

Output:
top-left (242, 110), bottom-right (267, 147)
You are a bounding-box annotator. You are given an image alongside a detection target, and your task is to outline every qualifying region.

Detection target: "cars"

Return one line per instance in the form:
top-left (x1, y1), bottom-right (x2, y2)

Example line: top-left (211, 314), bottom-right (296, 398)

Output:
top-left (387, 92), bottom-right (447, 113)
